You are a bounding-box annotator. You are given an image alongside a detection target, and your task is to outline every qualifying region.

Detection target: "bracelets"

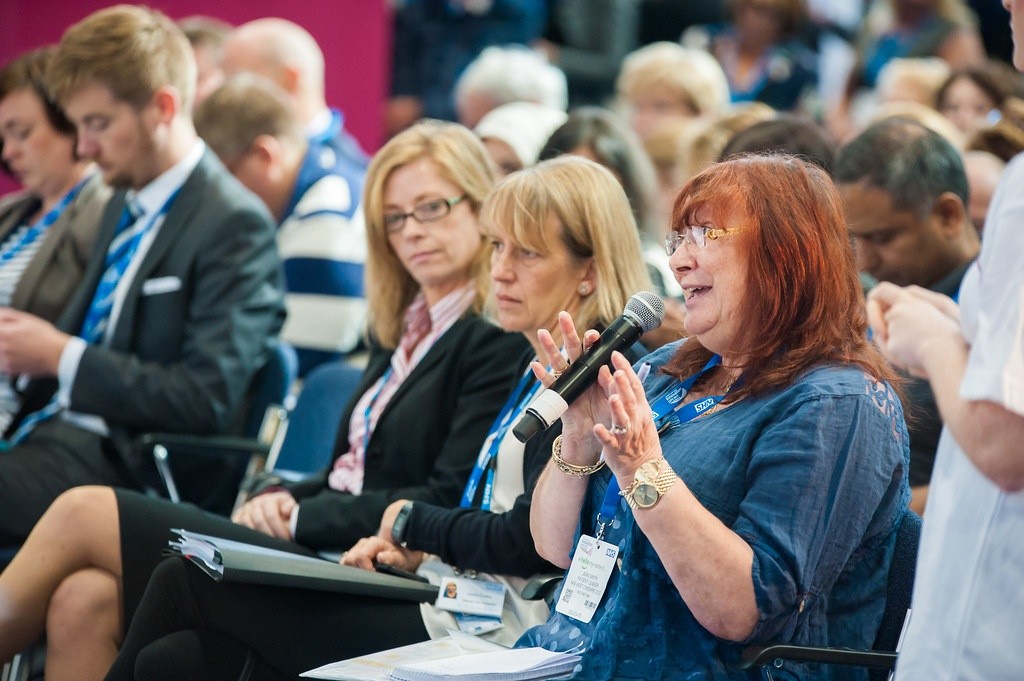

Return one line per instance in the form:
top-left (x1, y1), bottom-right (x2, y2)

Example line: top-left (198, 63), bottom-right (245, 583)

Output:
top-left (552, 434), bottom-right (605, 475)
top-left (618, 456), bottom-right (665, 495)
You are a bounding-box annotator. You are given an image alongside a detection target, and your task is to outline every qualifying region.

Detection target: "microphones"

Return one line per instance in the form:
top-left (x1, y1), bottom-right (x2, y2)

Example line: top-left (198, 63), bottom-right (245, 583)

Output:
top-left (512, 290), bottom-right (665, 443)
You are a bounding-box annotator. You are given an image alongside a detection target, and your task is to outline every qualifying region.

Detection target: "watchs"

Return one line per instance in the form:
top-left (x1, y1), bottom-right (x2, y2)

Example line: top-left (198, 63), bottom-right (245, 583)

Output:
top-left (391, 501), bottom-right (414, 541)
top-left (624, 465), bottom-right (677, 510)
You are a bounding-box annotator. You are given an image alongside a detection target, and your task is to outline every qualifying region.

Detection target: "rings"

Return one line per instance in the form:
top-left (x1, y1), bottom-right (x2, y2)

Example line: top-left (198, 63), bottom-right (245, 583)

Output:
top-left (554, 362), bottom-right (569, 377)
top-left (613, 421), bottom-right (630, 434)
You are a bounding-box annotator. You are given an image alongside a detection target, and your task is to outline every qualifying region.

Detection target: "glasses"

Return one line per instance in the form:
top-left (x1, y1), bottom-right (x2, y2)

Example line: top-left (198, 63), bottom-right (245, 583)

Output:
top-left (383, 194), bottom-right (469, 235)
top-left (664, 220), bottom-right (755, 255)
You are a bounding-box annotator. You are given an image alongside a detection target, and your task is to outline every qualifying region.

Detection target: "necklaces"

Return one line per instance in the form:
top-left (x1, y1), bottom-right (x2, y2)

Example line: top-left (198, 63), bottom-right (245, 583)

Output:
top-left (704, 380), bottom-right (716, 416)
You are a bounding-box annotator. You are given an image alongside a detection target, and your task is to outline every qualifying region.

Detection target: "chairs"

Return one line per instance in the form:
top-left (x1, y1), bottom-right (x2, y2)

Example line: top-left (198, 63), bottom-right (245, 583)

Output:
top-left (0, 334), bottom-right (925, 681)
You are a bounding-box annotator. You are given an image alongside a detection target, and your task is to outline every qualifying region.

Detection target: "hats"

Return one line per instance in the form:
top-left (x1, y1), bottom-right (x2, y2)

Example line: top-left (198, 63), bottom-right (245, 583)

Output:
top-left (478, 101), bottom-right (566, 175)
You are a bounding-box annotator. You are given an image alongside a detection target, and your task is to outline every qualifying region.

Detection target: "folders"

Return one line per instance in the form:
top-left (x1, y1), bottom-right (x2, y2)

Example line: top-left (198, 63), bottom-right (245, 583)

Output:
top-left (164, 530), bottom-right (439, 606)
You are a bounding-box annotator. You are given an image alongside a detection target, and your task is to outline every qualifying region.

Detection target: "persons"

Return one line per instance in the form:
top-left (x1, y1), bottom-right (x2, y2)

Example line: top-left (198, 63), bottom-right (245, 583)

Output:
top-left (0, 0), bottom-right (1024, 681)
top-left (445, 584), bottom-right (457, 598)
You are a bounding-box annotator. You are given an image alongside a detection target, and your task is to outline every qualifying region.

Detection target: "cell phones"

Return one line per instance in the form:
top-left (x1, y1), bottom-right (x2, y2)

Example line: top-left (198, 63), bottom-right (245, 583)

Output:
top-left (320, 552), bottom-right (430, 583)
